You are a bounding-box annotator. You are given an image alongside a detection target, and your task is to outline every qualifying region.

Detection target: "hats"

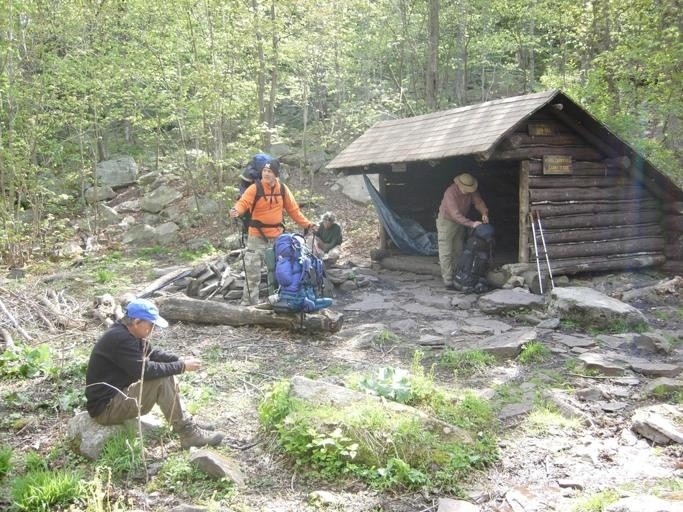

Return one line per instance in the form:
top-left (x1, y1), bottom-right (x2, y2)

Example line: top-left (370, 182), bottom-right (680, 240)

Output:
top-left (453, 173), bottom-right (478, 192)
top-left (127, 298), bottom-right (169, 328)
top-left (323, 213), bottom-right (335, 223)
top-left (261, 159), bottom-right (279, 177)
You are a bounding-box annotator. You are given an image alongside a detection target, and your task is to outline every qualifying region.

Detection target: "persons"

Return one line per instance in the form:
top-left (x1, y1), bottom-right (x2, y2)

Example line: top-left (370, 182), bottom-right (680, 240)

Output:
top-left (434, 172), bottom-right (489, 290)
top-left (306, 212), bottom-right (343, 266)
top-left (83, 298), bottom-right (223, 453)
top-left (226, 159), bottom-right (319, 308)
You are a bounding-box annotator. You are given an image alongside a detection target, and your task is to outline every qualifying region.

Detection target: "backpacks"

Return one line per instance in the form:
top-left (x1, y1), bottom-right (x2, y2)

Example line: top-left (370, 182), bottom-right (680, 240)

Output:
top-left (453, 223), bottom-right (497, 293)
top-left (275, 234), bottom-right (327, 312)
top-left (239, 154), bottom-right (272, 234)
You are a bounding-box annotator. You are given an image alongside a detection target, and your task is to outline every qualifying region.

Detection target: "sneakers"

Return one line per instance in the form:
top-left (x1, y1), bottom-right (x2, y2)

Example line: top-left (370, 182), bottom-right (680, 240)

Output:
top-left (444, 280), bottom-right (453, 287)
top-left (180, 422), bottom-right (225, 448)
